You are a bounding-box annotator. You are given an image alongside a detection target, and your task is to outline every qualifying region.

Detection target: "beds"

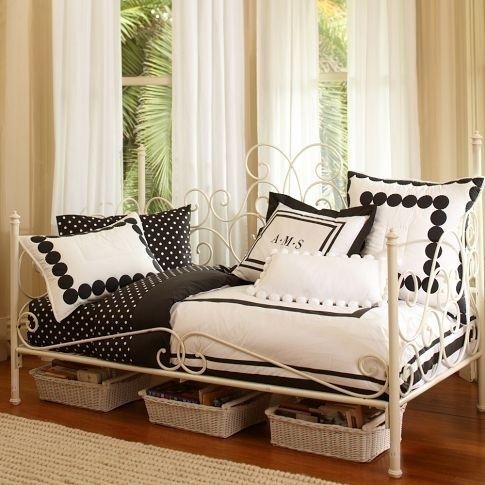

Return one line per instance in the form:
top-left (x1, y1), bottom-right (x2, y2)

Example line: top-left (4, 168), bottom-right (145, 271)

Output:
top-left (6, 133), bottom-right (483, 474)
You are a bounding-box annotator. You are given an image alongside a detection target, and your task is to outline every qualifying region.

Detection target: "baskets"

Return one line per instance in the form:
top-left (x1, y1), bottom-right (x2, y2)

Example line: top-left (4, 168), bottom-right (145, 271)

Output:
top-left (138, 378), bottom-right (271, 440)
top-left (28, 362), bottom-right (153, 413)
top-left (264, 403), bottom-right (409, 464)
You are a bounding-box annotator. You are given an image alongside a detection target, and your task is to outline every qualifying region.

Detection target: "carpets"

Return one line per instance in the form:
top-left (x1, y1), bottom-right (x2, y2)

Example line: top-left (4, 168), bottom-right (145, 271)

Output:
top-left (0, 411), bottom-right (343, 485)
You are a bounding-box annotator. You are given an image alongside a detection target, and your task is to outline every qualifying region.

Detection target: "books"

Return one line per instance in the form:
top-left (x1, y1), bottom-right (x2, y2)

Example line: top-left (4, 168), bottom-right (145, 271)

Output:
top-left (274, 396), bottom-right (409, 428)
top-left (38, 359), bottom-right (143, 386)
top-left (149, 378), bottom-right (267, 409)
